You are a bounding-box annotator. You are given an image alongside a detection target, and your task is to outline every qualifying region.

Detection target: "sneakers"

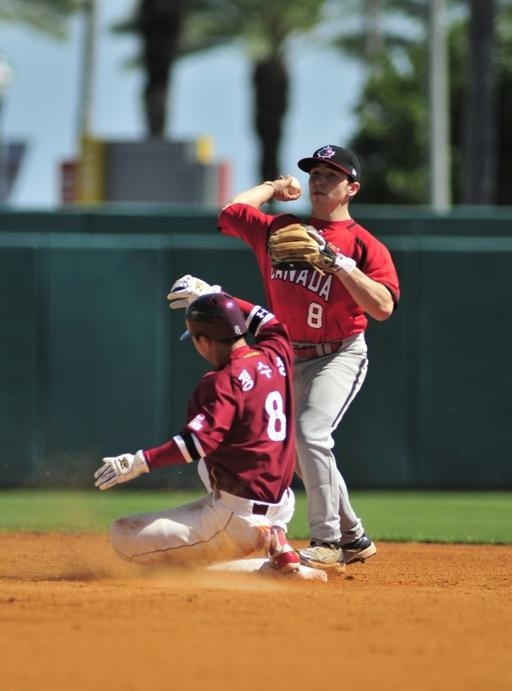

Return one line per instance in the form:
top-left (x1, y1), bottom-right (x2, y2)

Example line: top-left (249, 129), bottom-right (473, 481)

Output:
top-left (341, 533), bottom-right (377, 565)
top-left (264, 526), bottom-right (300, 575)
top-left (293, 538), bottom-right (346, 573)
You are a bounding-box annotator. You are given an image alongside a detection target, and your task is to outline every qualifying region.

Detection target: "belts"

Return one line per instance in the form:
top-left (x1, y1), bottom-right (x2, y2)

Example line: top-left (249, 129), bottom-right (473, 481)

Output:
top-left (210, 489), bottom-right (289, 515)
top-left (293, 341), bottom-right (342, 359)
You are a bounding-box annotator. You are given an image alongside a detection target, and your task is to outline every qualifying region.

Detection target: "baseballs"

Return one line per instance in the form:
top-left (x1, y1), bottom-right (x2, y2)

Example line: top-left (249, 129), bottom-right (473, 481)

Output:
top-left (288, 177), bottom-right (300, 194)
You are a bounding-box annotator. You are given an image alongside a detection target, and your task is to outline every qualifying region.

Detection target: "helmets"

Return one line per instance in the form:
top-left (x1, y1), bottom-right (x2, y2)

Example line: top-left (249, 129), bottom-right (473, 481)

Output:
top-left (179, 293), bottom-right (248, 342)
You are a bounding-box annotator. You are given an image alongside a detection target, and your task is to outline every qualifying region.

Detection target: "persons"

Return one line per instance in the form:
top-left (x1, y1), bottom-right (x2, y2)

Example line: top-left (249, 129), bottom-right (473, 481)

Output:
top-left (217, 144), bottom-right (400, 575)
top-left (93, 270), bottom-right (331, 583)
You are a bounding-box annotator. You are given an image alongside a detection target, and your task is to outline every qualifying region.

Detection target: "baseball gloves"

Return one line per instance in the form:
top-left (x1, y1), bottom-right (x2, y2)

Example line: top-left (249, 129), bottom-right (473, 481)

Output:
top-left (268, 223), bottom-right (340, 275)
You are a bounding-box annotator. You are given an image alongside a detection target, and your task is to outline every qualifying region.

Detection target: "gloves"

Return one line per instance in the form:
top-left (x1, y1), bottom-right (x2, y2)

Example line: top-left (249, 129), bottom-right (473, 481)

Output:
top-left (166, 274), bottom-right (222, 309)
top-left (94, 449), bottom-right (150, 491)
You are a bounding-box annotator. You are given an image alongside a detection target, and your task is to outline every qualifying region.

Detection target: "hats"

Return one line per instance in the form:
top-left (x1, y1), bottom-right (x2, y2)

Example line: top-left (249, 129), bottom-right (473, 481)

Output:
top-left (297, 145), bottom-right (361, 182)
